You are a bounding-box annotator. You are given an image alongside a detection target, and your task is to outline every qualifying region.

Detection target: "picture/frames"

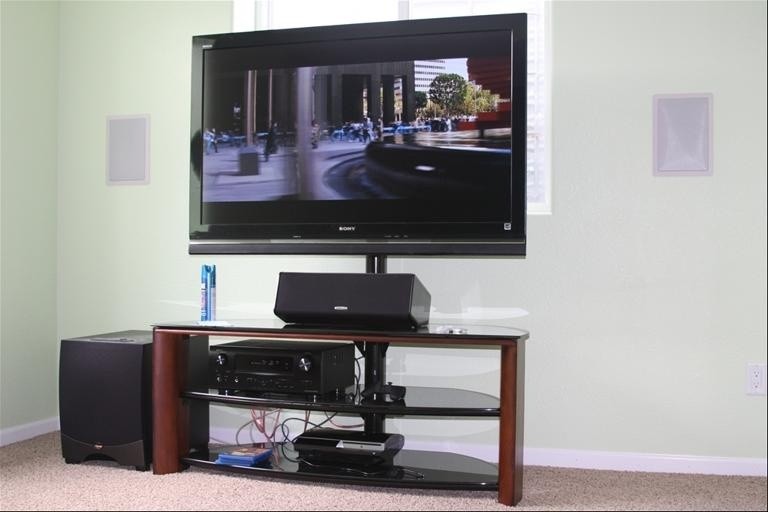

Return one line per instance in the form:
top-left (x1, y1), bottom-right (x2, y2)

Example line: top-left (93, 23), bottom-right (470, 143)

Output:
top-left (653, 92), bottom-right (713, 174)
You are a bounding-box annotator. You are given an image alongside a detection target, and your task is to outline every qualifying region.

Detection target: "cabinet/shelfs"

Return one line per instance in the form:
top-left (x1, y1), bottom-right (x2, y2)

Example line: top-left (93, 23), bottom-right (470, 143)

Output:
top-left (152, 323), bottom-right (528, 507)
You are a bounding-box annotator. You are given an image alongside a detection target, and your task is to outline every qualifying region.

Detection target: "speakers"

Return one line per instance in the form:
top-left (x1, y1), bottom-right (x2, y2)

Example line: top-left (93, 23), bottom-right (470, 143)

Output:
top-left (273, 272), bottom-right (431, 330)
top-left (59, 331), bottom-right (209, 472)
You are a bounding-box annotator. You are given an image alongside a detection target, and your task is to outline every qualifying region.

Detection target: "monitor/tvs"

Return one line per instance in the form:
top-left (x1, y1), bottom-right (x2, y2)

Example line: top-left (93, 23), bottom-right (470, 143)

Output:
top-left (189, 12), bottom-right (528, 273)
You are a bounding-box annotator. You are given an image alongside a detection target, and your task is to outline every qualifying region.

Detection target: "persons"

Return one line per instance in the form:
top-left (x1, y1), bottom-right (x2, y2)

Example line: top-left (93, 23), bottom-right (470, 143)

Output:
top-left (363, 117), bottom-right (384, 144)
top-left (203, 128), bottom-right (218, 154)
top-left (264, 121), bottom-right (279, 162)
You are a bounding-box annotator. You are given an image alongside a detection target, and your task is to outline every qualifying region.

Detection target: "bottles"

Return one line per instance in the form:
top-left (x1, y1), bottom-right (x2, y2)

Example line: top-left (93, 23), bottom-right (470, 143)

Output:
top-left (200, 264), bottom-right (217, 321)
